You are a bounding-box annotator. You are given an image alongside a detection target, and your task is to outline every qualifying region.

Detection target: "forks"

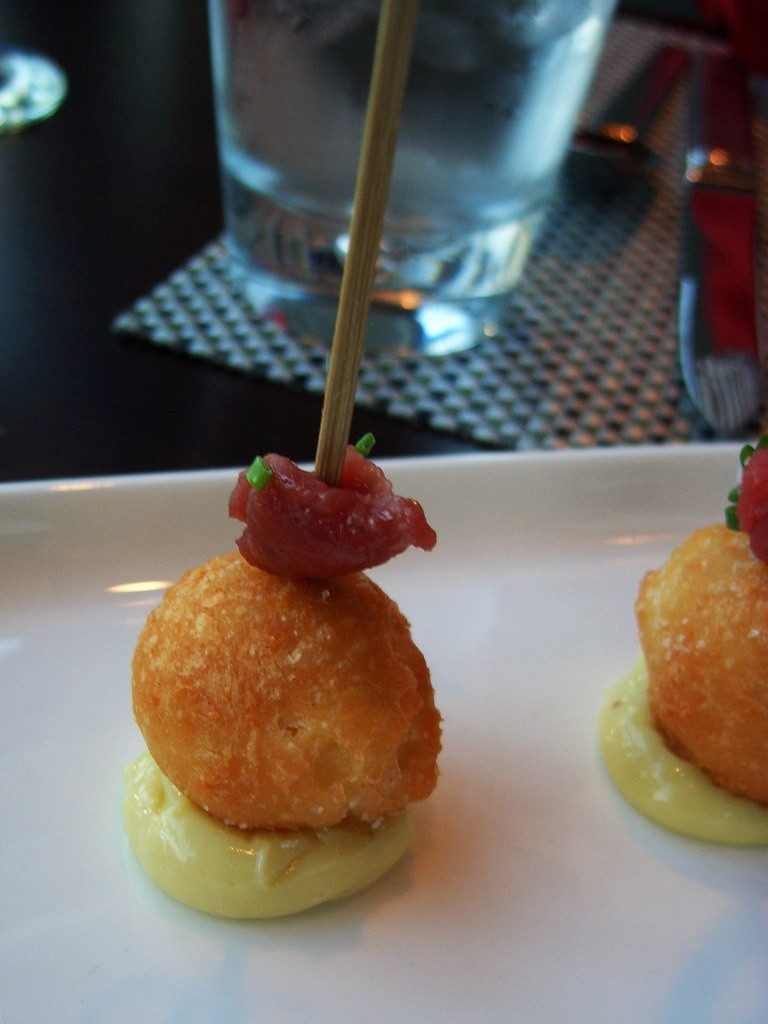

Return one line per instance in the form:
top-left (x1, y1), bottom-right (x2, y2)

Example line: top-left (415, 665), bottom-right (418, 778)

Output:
top-left (529, 40), bottom-right (706, 263)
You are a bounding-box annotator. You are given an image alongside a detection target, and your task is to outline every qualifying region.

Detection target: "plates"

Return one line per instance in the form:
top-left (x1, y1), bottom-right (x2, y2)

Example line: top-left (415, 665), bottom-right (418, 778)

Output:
top-left (0, 437), bottom-right (768, 1022)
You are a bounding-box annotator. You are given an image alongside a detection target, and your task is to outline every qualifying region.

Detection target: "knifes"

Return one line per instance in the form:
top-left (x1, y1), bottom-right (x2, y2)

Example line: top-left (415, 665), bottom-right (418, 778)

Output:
top-left (676, 48), bottom-right (763, 430)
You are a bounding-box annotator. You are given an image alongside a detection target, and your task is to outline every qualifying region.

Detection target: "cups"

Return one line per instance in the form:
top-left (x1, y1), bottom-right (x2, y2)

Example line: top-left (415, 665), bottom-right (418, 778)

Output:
top-left (194, 0), bottom-right (628, 361)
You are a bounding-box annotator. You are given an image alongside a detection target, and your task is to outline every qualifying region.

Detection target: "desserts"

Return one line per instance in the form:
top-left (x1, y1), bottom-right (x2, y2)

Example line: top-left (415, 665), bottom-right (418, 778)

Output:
top-left (120, 430), bottom-right (444, 917)
top-left (593, 429), bottom-right (768, 851)
